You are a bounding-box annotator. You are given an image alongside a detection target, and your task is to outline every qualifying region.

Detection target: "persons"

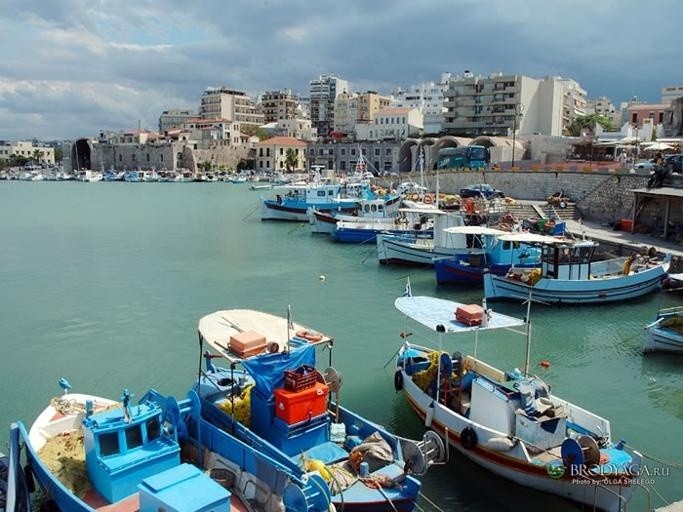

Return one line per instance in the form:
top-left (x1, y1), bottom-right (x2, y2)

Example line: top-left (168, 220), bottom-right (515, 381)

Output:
top-left (619, 148), bottom-right (626, 168)
top-left (646, 152), bottom-right (666, 189)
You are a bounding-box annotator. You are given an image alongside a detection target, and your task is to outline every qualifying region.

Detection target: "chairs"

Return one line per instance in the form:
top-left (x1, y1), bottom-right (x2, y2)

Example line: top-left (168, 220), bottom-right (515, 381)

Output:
top-left (439, 374), bottom-right (464, 414)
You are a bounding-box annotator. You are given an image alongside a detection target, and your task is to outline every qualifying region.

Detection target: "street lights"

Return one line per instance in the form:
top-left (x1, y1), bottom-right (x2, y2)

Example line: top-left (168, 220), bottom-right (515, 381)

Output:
top-left (510, 101), bottom-right (525, 170)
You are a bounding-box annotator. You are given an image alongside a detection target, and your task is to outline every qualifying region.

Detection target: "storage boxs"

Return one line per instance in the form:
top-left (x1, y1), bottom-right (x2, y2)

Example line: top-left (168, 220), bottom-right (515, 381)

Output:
top-left (274, 381), bottom-right (329, 425)
top-left (516, 410), bottom-right (566, 452)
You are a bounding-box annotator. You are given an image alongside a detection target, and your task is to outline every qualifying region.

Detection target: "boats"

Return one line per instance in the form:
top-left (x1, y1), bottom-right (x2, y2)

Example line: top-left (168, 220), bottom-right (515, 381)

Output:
top-left (431, 223), bottom-right (565, 285)
top-left (17, 378), bottom-right (333, 512)
top-left (376, 183), bottom-right (527, 270)
top-left (644, 308), bottom-right (682, 354)
top-left (482, 231), bottom-right (672, 308)
top-left (304, 179), bottom-right (426, 233)
top-left (393, 276), bottom-right (642, 511)
top-left (192, 309), bottom-right (445, 512)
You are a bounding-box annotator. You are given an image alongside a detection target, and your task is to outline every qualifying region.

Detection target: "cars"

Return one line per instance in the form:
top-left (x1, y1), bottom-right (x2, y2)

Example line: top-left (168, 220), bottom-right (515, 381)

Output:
top-left (633, 157), bottom-right (667, 171)
top-left (652, 155), bottom-right (682, 174)
top-left (362, 171), bottom-right (374, 179)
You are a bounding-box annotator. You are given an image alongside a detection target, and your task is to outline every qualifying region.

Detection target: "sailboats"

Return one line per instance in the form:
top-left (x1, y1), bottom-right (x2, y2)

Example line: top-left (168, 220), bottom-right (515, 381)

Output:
top-left (333, 144), bottom-right (446, 249)
top-left (257, 146), bottom-right (391, 224)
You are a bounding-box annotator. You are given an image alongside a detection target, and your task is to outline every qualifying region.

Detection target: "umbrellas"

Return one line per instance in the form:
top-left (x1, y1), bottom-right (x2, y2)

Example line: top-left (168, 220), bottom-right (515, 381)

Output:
top-left (643, 140), bottom-right (676, 155)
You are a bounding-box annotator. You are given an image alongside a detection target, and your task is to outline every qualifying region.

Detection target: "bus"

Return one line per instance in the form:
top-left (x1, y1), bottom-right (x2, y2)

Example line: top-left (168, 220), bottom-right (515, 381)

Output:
top-left (436, 145), bottom-right (488, 170)
